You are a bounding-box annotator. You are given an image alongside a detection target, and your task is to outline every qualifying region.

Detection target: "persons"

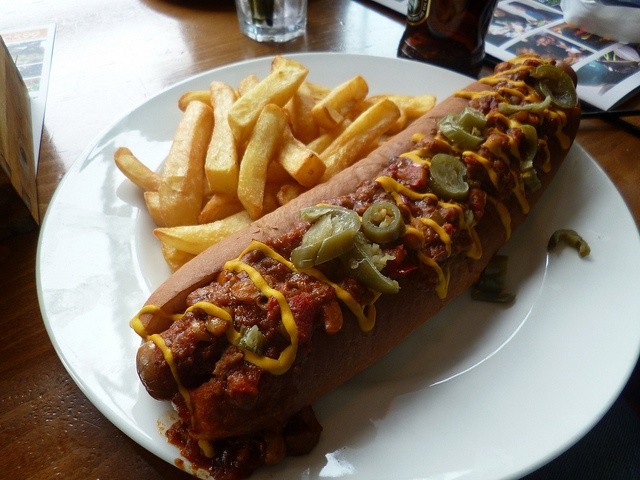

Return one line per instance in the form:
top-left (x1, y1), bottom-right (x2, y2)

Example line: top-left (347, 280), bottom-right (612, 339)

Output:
top-left (536, 34), bottom-right (587, 64)
top-left (509, 2), bottom-right (563, 27)
top-left (493, 8), bottom-right (533, 33)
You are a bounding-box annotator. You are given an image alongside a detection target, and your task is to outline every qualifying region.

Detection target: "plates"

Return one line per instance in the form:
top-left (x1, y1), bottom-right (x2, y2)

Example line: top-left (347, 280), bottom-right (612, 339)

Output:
top-left (34, 51), bottom-right (640, 480)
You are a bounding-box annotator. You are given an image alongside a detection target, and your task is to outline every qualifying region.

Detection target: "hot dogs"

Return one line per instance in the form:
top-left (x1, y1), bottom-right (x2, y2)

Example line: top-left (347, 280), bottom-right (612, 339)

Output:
top-left (131, 53), bottom-right (579, 478)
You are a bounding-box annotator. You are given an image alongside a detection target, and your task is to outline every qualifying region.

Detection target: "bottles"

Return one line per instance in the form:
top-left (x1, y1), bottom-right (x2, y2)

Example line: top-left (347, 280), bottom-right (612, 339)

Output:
top-left (395, 0), bottom-right (501, 78)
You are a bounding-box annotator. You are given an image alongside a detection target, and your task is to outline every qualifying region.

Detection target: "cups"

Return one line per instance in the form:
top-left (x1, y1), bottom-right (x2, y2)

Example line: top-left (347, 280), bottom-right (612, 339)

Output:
top-left (239, 0), bottom-right (308, 43)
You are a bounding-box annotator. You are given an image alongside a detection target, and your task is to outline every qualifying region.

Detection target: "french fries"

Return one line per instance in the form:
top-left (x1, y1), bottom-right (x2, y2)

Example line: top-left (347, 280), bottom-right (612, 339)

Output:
top-left (113, 53), bottom-right (437, 271)
